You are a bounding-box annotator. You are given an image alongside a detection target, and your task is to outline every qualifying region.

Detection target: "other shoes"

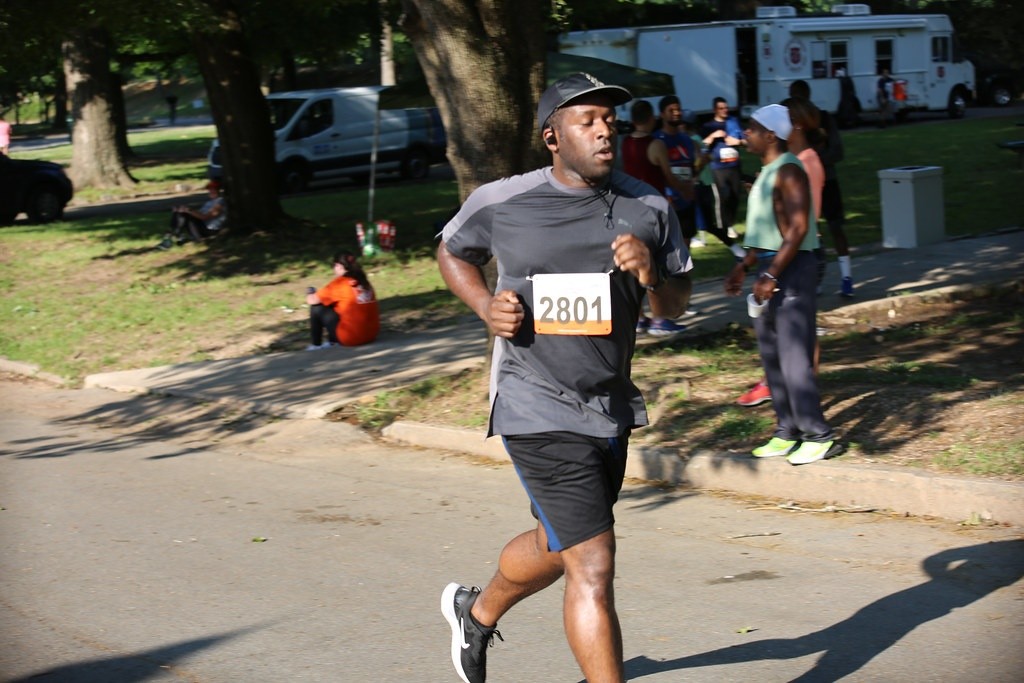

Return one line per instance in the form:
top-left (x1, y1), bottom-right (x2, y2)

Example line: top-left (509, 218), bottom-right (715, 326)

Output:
top-left (840, 276), bottom-right (855, 296)
top-left (683, 303), bottom-right (700, 316)
top-left (305, 342), bottom-right (337, 351)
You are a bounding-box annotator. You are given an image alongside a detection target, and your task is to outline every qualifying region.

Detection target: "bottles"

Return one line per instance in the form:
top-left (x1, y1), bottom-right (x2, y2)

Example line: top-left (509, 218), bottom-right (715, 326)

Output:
top-left (355, 219), bottom-right (395, 255)
top-left (664, 187), bottom-right (674, 204)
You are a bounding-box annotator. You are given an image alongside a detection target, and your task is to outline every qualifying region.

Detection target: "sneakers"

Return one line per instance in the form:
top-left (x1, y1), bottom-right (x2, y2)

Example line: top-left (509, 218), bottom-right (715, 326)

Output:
top-left (440, 581), bottom-right (505, 683)
top-left (636, 316), bottom-right (688, 336)
top-left (816, 284), bottom-right (825, 298)
top-left (752, 437), bottom-right (801, 457)
top-left (735, 378), bottom-right (772, 406)
top-left (786, 440), bottom-right (846, 465)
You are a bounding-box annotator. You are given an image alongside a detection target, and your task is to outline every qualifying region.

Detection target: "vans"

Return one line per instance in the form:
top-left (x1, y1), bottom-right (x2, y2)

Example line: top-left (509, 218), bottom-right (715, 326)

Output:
top-left (206, 85), bottom-right (448, 195)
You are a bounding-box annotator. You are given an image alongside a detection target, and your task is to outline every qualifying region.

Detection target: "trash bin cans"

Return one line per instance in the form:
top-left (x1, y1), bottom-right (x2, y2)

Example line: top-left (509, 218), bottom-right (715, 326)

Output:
top-left (878, 166), bottom-right (946, 248)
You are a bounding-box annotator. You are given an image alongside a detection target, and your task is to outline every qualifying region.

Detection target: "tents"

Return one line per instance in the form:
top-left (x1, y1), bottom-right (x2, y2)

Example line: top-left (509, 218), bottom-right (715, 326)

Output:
top-left (367, 51), bottom-right (676, 222)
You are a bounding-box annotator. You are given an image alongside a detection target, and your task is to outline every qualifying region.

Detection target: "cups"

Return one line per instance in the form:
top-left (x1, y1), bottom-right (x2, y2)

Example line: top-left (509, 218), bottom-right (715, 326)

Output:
top-left (746, 293), bottom-right (766, 318)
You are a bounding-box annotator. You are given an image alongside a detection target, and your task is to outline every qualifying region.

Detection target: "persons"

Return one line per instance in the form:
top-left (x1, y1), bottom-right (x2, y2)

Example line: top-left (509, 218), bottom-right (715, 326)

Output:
top-left (156, 179), bottom-right (228, 250)
top-left (621, 79), bottom-right (853, 335)
top-left (306, 244), bottom-right (380, 350)
top-left (724, 105), bottom-right (835, 464)
top-left (836, 67), bottom-right (862, 112)
top-left (876, 68), bottom-right (895, 113)
top-left (0, 113), bottom-right (10, 155)
top-left (437, 71), bottom-right (693, 683)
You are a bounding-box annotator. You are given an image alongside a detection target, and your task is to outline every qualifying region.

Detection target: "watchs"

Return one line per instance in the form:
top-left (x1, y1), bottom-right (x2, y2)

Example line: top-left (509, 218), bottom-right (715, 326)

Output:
top-left (640, 263), bottom-right (669, 291)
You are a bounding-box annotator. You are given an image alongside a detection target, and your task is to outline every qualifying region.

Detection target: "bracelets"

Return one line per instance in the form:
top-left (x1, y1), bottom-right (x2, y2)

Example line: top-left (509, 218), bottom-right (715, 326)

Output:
top-left (760, 272), bottom-right (781, 293)
top-left (740, 140), bottom-right (742, 145)
top-left (736, 258), bottom-right (750, 273)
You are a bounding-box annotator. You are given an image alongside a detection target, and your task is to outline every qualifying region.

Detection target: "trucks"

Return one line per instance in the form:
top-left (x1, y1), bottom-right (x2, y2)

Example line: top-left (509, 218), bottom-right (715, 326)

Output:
top-left (555, 3), bottom-right (976, 126)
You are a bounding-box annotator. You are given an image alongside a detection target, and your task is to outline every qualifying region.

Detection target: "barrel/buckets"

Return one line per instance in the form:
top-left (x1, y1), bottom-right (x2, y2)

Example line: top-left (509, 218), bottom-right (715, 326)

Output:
top-left (894, 80), bottom-right (907, 100)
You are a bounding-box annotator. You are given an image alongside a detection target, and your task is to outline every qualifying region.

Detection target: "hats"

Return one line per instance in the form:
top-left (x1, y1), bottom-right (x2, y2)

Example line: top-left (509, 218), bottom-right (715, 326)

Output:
top-left (751, 104), bottom-right (794, 141)
top-left (536, 71), bottom-right (633, 134)
top-left (206, 181), bottom-right (221, 189)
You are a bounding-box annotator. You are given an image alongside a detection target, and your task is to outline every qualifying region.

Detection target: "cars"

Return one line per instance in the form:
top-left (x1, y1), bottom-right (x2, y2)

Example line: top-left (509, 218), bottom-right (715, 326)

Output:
top-left (955, 52), bottom-right (1024, 107)
top-left (0, 151), bottom-right (74, 228)
